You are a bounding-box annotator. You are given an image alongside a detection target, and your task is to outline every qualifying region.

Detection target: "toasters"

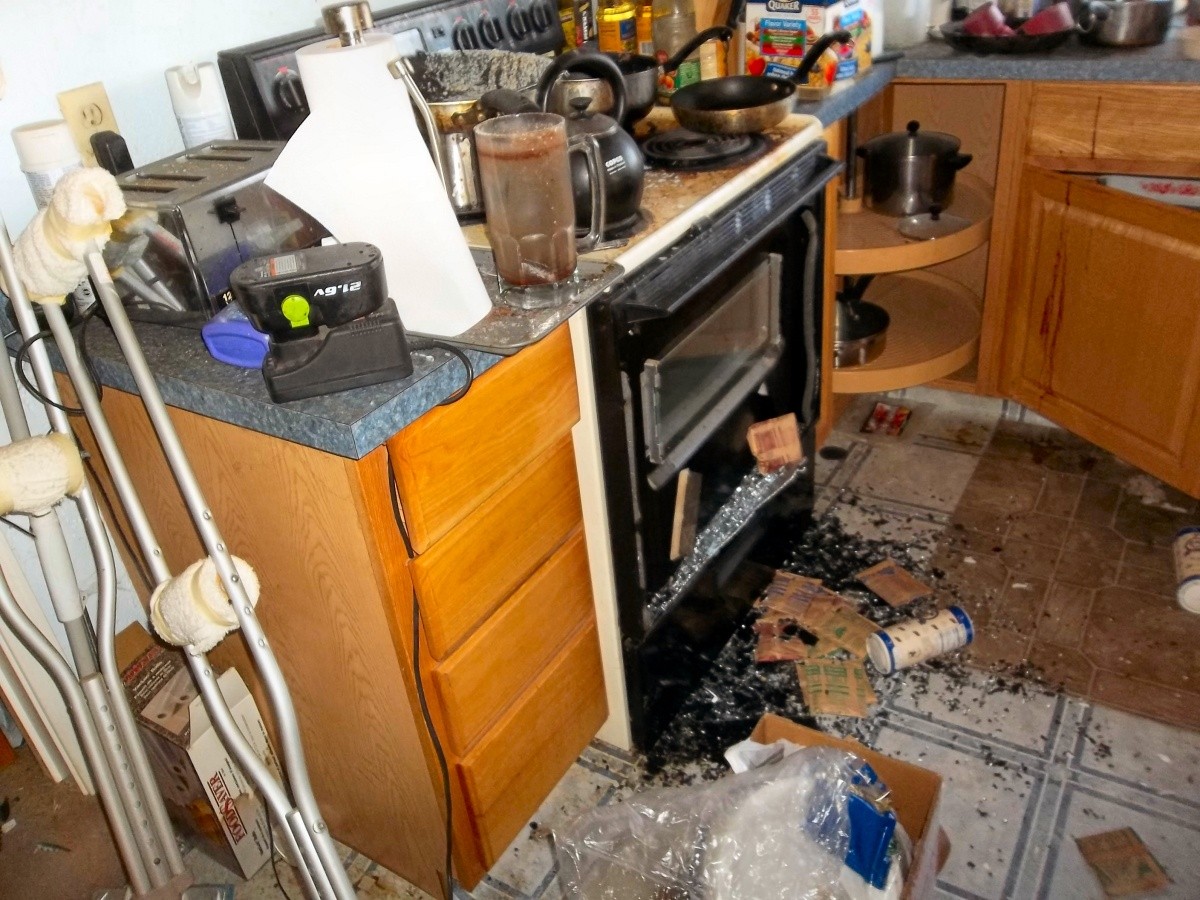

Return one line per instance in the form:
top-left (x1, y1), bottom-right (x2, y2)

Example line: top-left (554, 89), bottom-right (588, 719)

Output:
top-left (95, 135), bottom-right (330, 324)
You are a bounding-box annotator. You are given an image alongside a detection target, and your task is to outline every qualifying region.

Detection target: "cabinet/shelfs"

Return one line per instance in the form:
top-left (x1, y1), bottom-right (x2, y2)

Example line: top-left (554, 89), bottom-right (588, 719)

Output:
top-left (809, 81), bottom-right (1200, 500)
top-left (55, 317), bottom-right (612, 900)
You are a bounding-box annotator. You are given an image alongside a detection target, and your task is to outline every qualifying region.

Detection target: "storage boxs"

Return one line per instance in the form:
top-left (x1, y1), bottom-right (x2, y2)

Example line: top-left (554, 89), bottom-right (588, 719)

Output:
top-left (114, 620), bottom-right (288, 881)
top-left (691, 711), bottom-right (942, 900)
top-left (745, 1), bottom-right (882, 101)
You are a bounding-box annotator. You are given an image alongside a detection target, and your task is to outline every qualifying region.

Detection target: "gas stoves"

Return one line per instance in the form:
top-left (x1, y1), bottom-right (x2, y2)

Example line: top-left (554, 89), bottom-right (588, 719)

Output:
top-left (219, 0), bottom-right (822, 275)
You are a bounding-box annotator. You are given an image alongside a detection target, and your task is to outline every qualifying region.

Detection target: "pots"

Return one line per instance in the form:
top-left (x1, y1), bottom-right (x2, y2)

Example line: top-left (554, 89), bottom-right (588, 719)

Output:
top-left (833, 274), bottom-right (891, 370)
top-left (669, 30), bottom-right (852, 135)
top-left (1065, 0), bottom-right (1175, 47)
top-left (550, 25), bottom-right (733, 130)
top-left (854, 120), bottom-right (972, 220)
top-left (411, 47), bottom-right (553, 216)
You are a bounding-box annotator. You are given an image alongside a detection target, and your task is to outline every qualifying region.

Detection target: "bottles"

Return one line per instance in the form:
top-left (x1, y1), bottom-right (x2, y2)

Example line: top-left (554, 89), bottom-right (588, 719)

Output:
top-left (596, 1), bottom-right (726, 105)
top-left (201, 297), bottom-right (269, 368)
top-left (163, 59), bottom-right (235, 150)
top-left (11, 119), bottom-right (100, 312)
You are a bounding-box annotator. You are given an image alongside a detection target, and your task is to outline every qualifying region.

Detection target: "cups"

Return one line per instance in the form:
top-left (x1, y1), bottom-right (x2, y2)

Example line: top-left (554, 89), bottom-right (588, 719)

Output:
top-left (473, 111), bottom-right (606, 309)
top-left (865, 604), bottom-right (975, 674)
top-left (1172, 526), bottom-right (1200, 615)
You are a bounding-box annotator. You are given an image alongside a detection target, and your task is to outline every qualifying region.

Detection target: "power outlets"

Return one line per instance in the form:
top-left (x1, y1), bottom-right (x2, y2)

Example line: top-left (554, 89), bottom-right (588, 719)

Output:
top-left (56, 81), bottom-right (120, 169)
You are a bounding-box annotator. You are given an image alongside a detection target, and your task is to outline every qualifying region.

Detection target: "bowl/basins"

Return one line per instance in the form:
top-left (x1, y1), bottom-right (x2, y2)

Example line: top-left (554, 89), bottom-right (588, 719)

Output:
top-left (941, 16), bottom-right (1076, 55)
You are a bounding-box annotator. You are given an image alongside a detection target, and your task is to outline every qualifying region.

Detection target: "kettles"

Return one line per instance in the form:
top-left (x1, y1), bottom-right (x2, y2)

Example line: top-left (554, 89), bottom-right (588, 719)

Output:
top-left (535, 48), bottom-right (644, 230)
